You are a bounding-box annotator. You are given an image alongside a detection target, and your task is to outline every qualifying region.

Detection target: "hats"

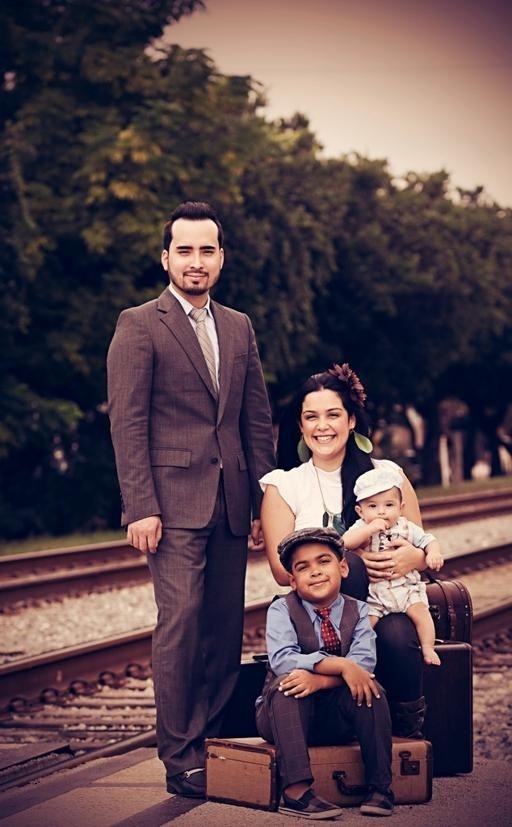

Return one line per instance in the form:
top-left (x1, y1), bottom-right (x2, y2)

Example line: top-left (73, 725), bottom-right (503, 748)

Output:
top-left (277, 527), bottom-right (345, 575)
top-left (353, 468), bottom-right (404, 502)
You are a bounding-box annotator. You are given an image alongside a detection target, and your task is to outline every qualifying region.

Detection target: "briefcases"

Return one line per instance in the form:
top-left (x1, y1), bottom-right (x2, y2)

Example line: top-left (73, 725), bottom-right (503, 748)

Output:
top-left (203, 737), bottom-right (433, 812)
top-left (223, 655), bottom-right (269, 737)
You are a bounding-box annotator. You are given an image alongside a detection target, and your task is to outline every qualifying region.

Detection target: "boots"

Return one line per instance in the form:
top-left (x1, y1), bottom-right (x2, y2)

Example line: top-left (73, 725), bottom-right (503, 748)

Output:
top-left (390, 696), bottom-right (428, 738)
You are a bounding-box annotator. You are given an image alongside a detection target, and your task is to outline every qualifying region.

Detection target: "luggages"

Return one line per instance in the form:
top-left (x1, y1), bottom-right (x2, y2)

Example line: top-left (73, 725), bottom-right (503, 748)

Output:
top-left (424, 569), bottom-right (474, 775)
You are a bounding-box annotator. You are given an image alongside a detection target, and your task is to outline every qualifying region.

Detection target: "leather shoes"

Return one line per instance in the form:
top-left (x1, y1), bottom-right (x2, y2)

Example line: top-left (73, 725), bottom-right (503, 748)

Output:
top-left (167, 768), bottom-right (204, 798)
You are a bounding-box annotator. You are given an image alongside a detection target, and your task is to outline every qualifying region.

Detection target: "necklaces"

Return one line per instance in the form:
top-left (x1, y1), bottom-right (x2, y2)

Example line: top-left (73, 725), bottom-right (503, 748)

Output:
top-left (313, 466), bottom-right (344, 527)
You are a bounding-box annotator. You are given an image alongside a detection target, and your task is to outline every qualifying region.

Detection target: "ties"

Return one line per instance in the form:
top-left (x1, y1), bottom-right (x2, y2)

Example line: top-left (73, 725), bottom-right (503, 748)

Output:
top-left (190, 309), bottom-right (218, 394)
top-left (314, 607), bottom-right (341, 656)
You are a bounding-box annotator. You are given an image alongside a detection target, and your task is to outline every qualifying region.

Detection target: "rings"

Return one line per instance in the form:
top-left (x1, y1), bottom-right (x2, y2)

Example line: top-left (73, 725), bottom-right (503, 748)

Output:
top-left (390, 567), bottom-right (394, 573)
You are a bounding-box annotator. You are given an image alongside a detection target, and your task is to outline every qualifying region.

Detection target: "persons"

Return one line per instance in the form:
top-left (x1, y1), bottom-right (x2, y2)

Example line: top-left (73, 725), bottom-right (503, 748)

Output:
top-left (340, 465), bottom-right (445, 666)
top-left (258, 360), bottom-right (429, 739)
top-left (106, 200), bottom-right (278, 800)
top-left (253, 527), bottom-right (397, 822)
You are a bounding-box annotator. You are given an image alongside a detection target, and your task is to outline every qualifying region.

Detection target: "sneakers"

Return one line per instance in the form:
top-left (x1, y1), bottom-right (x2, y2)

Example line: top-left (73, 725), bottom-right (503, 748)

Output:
top-left (278, 789), bottom-right (342, 819)
top-left (360, 790), bottom-right (395, 816)
